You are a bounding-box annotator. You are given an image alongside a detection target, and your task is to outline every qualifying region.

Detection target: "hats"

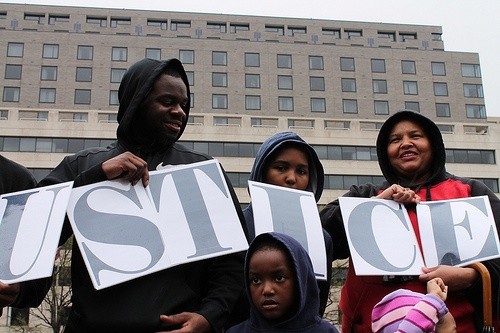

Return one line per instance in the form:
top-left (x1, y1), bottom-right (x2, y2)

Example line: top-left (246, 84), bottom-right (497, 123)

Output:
top-left (371, 290), bottom-right (436, 333)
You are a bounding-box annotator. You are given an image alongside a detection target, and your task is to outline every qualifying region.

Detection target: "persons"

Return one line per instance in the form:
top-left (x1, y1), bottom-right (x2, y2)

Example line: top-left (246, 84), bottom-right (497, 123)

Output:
top-left (244, 132), bottom-right (334, 318)
top-left (320, 111), bottom-right (500, 333)
top-left (372, 278), bottom-right (457, 333)
top-left (0, 155), bottom-right (52, 309)
top-left (38, 58), bottom-right (249, 333)
top-left (225, 233), bottom-right (340, 333)
